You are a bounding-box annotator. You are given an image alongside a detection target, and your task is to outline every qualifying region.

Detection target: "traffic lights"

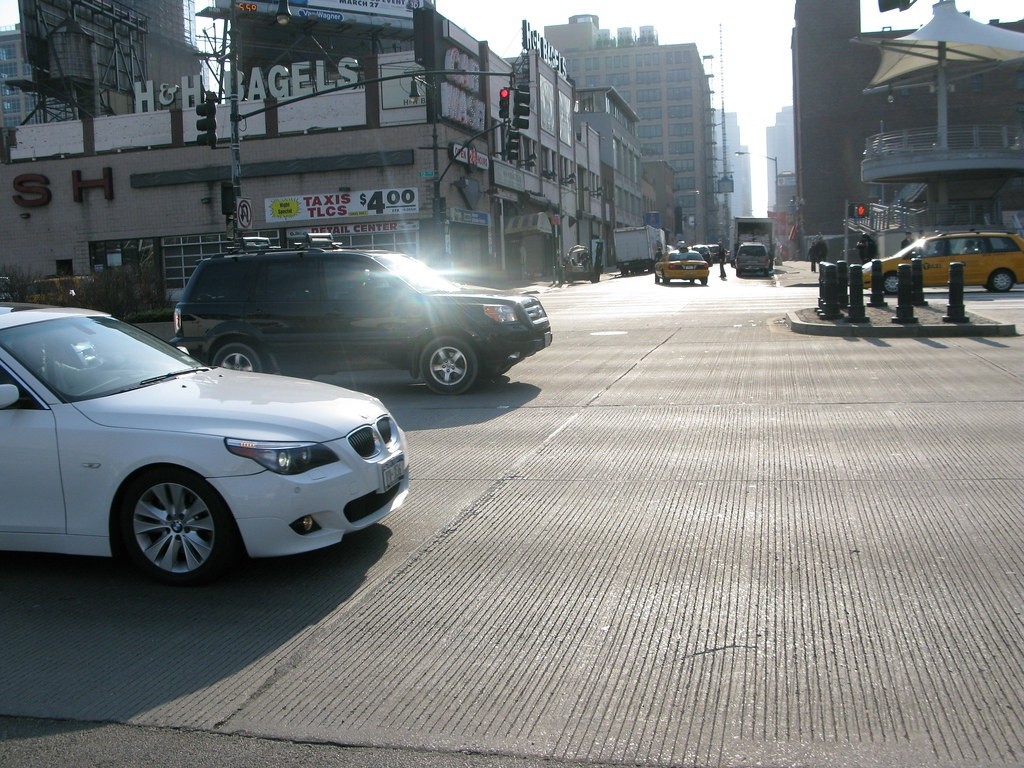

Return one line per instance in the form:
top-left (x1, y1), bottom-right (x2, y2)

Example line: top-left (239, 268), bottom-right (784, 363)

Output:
top-left (499, 87), bottom-right (510, 120)
top-left (195, 92), bottom-right (218, 149)
top-left (513, 87), bottom-right (532, 131)
top-left (855, 203), bottom-right (870, 218)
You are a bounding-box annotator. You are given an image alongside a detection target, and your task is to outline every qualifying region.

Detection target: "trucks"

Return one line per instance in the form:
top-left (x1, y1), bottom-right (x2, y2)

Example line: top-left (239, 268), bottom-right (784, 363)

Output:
top-left (732, 217), bottom-right (776, 264)
top-left (612, 224), bottom-right (667, 277)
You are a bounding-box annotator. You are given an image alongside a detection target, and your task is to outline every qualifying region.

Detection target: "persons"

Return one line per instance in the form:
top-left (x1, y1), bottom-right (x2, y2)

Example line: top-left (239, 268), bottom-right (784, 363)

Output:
top-left (807, 227), bottom-right (978, 272)
top-left (654, 247), bottom-right (663, 262)
top-left (718, 241), bottom-right (728, 278)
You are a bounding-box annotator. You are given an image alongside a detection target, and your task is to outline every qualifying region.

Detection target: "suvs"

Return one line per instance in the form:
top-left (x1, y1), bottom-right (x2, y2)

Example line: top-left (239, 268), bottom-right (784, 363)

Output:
top-left (169, 232), bottom-right (553, 398)
top-left (862, 228), bottom-right (1024, 293)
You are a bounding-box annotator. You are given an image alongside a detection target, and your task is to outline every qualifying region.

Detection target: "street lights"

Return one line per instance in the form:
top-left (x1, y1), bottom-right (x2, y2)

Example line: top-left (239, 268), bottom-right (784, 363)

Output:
top-left (735, 150), bottom-right (779, 221)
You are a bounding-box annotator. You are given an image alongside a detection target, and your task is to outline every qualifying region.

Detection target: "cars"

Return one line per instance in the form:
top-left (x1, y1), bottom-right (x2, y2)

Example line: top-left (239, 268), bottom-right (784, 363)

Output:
top-left (654, 249), bottom-right (709, 286)
top-left (733, 242), bottom-right (772, 277)
top-left (1, 301), bottom-right (413, 588)
top-left (692, 243), bottom-right (731, 263)
